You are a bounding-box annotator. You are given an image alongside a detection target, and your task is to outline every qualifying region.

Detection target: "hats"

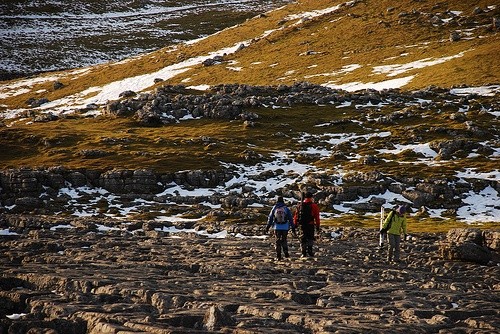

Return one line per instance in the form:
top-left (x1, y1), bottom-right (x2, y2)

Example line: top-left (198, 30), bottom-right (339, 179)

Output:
top-left (277, 197), bottom-right (284, 203)
top-left (304, 192), bottom-right (313, 197)
top-left (399, 206), bottom-right (406, 215)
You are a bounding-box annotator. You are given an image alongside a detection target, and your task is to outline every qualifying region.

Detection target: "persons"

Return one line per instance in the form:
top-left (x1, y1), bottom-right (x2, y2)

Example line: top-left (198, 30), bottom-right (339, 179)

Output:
top-left (379, 203), bottom-right (409, 263)
top-left (293, 192), bottom-right (321, 258)
top-left (265, 196), bottom-right (295, 259)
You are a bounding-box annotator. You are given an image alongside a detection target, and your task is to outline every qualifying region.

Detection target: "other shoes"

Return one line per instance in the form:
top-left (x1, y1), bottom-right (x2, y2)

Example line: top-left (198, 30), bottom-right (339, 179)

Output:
top-left (301, 253), bottom-right (306, 258)
top-left (387, 258), bottom-right (401, 261)
top-left (277, 253), bottom-right (280, 258)
top-left (284, 251), bottom-right (289, 258)
top-left (308, 251), bottom-right (314, 257)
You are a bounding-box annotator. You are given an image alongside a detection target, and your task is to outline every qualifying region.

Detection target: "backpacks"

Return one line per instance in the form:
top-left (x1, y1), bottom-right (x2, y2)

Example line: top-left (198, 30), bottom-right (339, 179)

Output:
top-left (298, 204), bottom-right (313, 229)
top-left (274, 207), bottom-right (289, 223)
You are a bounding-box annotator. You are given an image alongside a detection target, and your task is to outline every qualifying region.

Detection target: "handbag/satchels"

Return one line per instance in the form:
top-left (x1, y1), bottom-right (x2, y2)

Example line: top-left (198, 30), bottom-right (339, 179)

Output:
top-left (381, 223), bottom-right (391, 233)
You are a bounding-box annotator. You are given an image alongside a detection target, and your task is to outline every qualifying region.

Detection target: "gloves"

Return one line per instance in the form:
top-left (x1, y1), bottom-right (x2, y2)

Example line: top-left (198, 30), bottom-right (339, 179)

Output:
top-left (404, 234), bottom-right (407, 240)
top-left (380, 228), bottom-right (384, 234)
top-left (316, 227), bottom-right (319, 229)
top-left (292, 228), bottom-right (295, 231)
top-left (265, 226), bottom-right (269, 233)
